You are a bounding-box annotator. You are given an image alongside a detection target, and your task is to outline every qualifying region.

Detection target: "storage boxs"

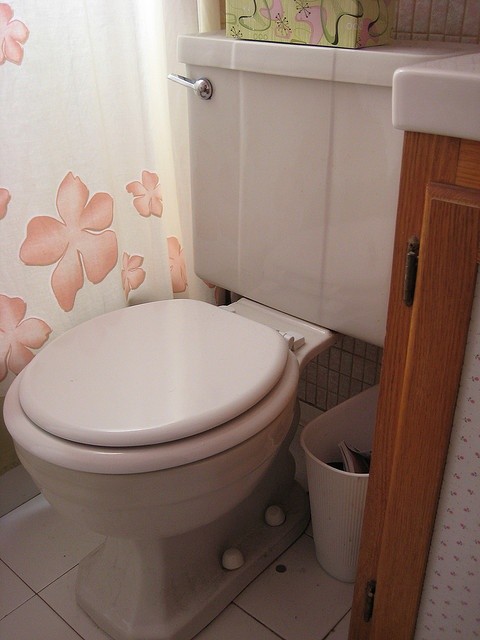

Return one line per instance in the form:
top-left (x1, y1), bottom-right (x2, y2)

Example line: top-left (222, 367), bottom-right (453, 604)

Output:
top-left (225, 0), bottom-right (400, 49)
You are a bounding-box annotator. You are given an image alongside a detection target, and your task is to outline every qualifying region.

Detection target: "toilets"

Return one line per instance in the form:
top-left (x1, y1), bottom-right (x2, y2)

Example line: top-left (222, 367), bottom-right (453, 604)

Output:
top-left (1, 35), bottom-right (480, 639)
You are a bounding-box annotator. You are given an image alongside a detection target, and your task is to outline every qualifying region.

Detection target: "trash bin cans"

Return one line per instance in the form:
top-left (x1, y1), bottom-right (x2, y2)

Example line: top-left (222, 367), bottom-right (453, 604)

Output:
top-left (299, 384), bottom-right (380, 583)
